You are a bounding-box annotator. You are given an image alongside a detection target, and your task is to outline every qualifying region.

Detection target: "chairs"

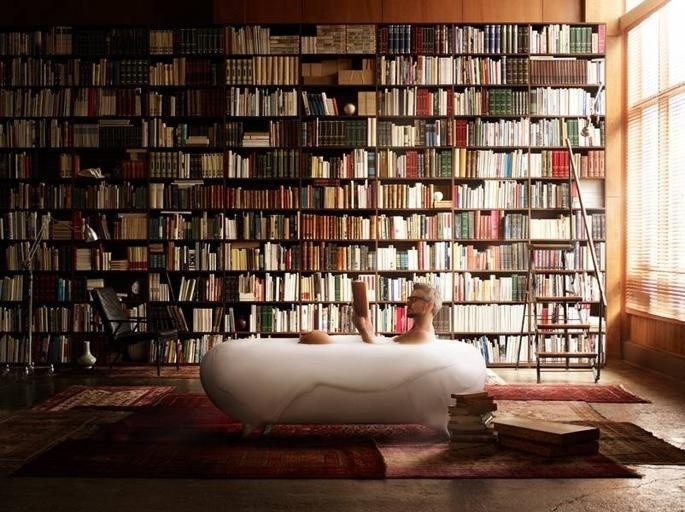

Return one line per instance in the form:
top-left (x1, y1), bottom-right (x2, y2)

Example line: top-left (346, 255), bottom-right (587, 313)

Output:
top-left (84, 274), bottom-right (188, 376)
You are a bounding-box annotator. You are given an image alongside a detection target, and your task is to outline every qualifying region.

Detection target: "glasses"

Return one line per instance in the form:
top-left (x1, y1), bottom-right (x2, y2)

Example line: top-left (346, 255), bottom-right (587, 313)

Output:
top-left (406, 297), bottom-right (425, 303)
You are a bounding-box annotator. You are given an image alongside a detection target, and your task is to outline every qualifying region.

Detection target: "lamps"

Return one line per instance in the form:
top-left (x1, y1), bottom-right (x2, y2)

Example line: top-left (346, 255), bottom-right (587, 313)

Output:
top-left (18, 210), bottom-right (103, 374)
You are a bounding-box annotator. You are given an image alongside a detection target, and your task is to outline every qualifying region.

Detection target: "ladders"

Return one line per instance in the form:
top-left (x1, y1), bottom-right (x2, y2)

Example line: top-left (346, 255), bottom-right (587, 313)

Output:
top-left (515, 138), bottom-right (607, 384)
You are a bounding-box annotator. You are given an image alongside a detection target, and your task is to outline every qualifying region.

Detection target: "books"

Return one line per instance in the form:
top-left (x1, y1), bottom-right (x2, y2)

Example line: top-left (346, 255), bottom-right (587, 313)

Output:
top-left (490, 414), bottom-right (601, 442)
top-left (0, 16), bottom-right (607, 370)
top-left (447, 389), bottom-right (498, 457)
top-left (497, 433), bottom-right (599, 460)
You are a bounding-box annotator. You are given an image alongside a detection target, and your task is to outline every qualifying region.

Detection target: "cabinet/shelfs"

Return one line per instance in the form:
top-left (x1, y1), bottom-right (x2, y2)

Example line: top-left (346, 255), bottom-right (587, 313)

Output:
top-left (0, 21), bottom-right (608, 366)
